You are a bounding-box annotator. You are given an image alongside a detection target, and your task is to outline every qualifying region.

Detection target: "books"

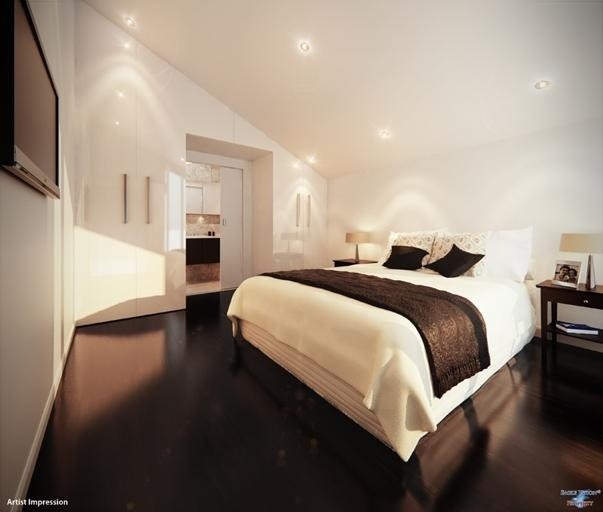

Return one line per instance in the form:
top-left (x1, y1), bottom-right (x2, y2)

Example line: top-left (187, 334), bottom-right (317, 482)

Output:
top-left (555, 320), bottom-right (599, 336)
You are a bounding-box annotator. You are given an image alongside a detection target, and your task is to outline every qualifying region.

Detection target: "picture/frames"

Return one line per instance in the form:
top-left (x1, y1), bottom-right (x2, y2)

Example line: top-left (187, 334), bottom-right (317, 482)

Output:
top-left (551, 259), bottom-right (584, 288)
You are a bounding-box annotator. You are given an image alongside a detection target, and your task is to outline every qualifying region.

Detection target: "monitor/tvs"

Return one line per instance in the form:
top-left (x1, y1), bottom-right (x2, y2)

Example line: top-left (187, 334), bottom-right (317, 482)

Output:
top-left (0, 0), bottom-right (60, 200)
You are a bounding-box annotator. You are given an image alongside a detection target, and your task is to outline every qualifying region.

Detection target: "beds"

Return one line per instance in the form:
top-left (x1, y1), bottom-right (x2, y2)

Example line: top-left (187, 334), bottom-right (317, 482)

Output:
top-left (235, 222), bottom-right (536, 456)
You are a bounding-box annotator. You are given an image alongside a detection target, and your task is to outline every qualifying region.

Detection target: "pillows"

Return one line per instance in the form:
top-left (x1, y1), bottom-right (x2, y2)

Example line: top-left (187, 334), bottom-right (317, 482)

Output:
top-left (377, 226), bottom-right (532, 282)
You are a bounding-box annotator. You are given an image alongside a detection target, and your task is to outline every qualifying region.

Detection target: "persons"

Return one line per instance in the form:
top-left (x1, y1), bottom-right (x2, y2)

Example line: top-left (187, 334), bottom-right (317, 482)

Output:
top-left (558, 265), bottom-right (569, 280)
top-left (566, 268), bottom-right (577, 283)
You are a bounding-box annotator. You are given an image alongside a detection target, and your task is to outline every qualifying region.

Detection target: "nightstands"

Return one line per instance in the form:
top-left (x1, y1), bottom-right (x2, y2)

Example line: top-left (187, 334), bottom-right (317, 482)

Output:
top-left (535, 279), bottom-right (602, 371)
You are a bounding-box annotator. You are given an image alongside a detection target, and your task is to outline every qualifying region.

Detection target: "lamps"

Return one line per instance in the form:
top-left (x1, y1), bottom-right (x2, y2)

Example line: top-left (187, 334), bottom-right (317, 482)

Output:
top-left (558, 233), bottom-right (603, 289)
top-left (345, 232), bottom-right (369, 262)
